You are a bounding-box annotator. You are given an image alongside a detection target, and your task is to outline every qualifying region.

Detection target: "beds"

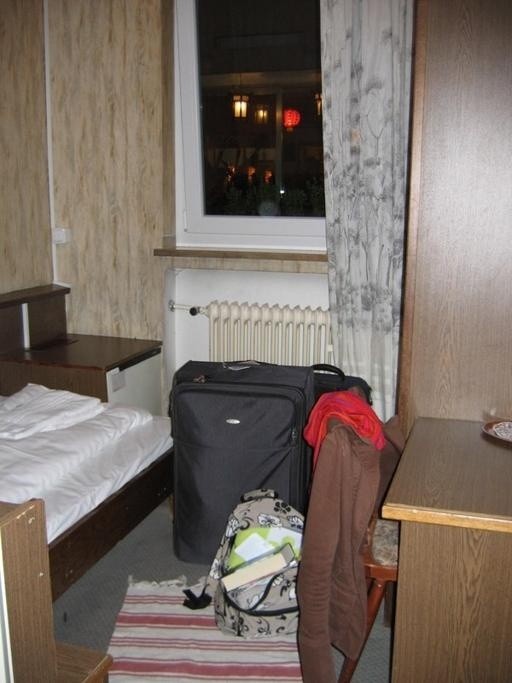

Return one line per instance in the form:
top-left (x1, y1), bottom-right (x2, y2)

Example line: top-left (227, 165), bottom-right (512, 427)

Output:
top-left (0, 377), bottom-right (175, 605)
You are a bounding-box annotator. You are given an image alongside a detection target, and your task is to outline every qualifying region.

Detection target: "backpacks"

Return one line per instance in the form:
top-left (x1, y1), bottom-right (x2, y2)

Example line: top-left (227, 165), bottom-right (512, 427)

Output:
top-left (180, 489), bottom-right (305, 641)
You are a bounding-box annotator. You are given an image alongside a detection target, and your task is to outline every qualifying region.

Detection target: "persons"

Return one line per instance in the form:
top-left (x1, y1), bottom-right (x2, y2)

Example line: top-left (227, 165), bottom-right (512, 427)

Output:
top-left (224, 164), bottom-right (277, 216)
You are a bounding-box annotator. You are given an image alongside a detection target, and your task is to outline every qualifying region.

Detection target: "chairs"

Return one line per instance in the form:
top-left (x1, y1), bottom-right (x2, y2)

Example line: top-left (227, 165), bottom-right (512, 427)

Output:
top-left (0, 496), bottom-right (113, 681)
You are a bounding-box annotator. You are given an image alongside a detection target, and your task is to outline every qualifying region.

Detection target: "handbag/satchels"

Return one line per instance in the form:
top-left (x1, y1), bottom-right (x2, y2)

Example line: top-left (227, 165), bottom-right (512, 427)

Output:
top-left (310, 363), bottom-right (372, 409)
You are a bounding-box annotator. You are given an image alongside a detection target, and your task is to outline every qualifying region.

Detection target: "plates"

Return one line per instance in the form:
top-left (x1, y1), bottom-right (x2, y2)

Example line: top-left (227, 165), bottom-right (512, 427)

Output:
top-left (480, 419), bottom-right (512, 443)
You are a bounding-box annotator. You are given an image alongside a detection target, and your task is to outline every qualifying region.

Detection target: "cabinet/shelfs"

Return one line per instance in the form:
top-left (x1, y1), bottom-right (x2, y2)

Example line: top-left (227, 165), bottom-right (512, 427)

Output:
top-left (391, 5), bottom-right (512, 428)
top-left (1, 285), bottom-right (163, 420)
top-left (385, 415), bottom-right (512, 683)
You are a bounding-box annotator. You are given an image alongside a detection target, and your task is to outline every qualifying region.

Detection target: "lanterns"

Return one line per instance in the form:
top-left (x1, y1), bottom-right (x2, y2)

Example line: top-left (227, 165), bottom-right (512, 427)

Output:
top-left (283, 109), bottom-right (301, 133)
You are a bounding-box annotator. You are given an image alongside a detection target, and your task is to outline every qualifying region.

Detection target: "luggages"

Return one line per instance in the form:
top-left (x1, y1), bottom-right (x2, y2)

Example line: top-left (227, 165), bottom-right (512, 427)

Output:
top-left (167, 359), bottom-right (314, 562)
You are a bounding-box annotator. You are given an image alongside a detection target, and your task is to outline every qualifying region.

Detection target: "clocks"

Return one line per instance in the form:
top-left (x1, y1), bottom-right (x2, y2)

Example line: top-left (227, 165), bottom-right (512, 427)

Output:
top-left (105, 572), bottom-right (307, 681)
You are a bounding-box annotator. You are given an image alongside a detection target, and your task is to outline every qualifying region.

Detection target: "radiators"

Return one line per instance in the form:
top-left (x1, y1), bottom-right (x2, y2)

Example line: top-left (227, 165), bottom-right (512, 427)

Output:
top-left (169, 301), bottom-right (336, 375)
